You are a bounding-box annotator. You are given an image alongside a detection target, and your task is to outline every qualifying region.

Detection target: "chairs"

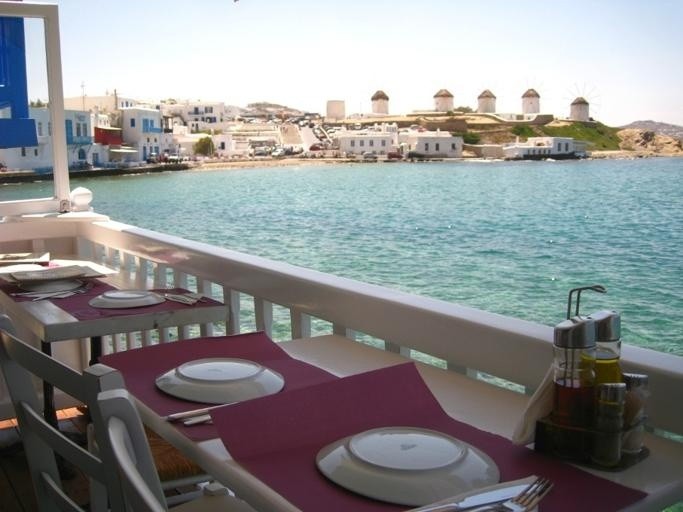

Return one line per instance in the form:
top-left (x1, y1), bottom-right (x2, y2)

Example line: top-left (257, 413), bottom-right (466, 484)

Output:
top-left (96, 388), bottom-right (252, 511)
top-left (2, 313), bottom-right (212, 512)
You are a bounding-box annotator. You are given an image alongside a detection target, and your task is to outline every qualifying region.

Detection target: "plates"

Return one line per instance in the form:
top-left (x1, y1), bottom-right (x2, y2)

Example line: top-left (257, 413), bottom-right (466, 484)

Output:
top-left (87, 288), bottom-right (166, 310)
top-left (154, 357), bottom-right (286, 405)
top-left (13, 278), bottom-right (85, 291)
top-left (314, 423), bottom-right (503, 507)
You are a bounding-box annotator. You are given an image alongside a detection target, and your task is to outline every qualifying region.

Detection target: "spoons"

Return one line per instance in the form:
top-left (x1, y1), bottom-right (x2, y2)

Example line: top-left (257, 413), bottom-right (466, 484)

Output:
top-left (32, 281), bottom-right (96, 302)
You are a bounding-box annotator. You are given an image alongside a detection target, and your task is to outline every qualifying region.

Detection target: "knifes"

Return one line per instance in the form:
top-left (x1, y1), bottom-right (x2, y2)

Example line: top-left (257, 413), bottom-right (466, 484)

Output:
top-left (401, 474), bottom-right (538, 512)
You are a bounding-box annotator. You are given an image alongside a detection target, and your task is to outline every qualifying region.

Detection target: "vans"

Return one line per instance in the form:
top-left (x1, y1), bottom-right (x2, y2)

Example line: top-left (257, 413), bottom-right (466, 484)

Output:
top-left (409, 125), bottom-right (419, 130)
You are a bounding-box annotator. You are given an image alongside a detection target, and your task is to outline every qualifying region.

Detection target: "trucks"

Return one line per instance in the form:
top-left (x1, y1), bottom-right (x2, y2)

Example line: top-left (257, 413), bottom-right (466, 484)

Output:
top-left (347, 123), bottom-right (363, 130)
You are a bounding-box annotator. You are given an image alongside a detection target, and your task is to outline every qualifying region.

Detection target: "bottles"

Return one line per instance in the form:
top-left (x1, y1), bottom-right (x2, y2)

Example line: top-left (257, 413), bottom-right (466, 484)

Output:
top-left (553, 308), bottom-right (649, 467)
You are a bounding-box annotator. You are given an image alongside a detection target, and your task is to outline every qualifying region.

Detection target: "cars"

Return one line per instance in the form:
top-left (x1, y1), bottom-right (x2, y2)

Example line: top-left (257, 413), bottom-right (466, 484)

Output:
top-left (145, 152), bottom-right (202, 164)
top-left (386, 151), bottom-right (403, 159)
top-left (407, 151), bottom-right (424, 158)
top-left (242, 115), bottom-right (335, 158)
top-left (342, 152), bottom-right (357, 158)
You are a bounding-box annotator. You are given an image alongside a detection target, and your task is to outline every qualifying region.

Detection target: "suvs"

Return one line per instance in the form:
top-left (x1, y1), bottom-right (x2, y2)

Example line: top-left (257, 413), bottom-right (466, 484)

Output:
top-left (362, 151), bottom-right (378, 159)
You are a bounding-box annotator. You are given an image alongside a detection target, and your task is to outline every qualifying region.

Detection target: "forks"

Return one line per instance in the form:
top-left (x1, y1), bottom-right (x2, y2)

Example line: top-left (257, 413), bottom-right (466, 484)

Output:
top-left (461, 476), bottom-right (554, 512)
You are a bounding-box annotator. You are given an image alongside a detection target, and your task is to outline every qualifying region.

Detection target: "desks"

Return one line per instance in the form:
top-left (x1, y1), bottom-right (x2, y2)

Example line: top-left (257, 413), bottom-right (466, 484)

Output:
top-left (1, 255), bottom-right (229, 480)
top-left (201, 335), bottom-right (681, 512)
top-left (0, 247), bottom-right (683, 511)
